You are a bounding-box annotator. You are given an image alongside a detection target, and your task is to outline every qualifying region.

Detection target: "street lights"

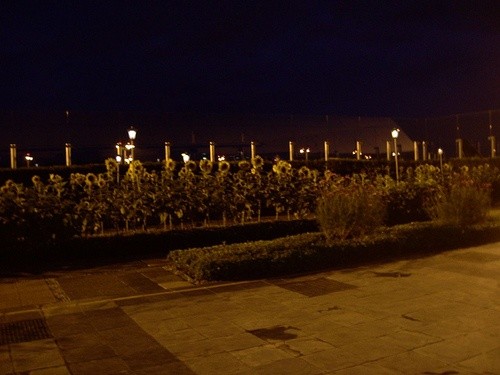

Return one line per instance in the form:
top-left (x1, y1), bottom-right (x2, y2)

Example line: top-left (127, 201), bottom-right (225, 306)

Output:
top-left (392, 129), bottom-right (401, 184)
top-left (127, 129), bottom-right (138, 187)
